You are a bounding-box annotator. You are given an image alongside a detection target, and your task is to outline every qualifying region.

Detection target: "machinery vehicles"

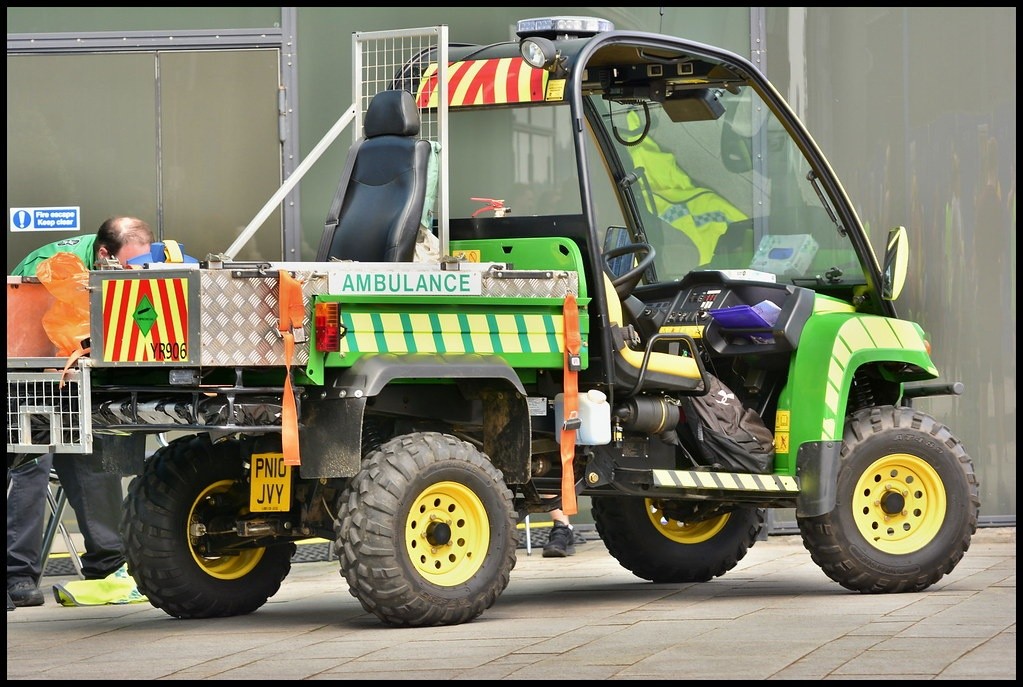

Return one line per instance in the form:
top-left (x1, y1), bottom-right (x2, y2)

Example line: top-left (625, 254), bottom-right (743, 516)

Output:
top-left (6, 16), bottom-right (981, 628)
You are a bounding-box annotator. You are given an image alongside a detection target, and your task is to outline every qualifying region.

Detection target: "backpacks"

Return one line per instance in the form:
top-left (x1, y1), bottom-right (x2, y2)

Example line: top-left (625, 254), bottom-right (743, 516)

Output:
top-left (685, 371), bottom-right (775, 474)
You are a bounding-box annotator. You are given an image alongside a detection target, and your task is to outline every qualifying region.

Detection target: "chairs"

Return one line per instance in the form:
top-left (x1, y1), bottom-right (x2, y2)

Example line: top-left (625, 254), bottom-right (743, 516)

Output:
top-left (314, 89), bottom-right (432, 262)
top-left (602, 272), bottom-right (712, 396)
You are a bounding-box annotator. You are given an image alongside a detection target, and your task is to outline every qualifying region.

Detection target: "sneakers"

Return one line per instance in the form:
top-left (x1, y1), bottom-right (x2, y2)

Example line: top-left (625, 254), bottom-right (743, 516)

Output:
top-left (9, 582), bottom-right (45, 606)
top-left (542, 519), bottom-right (576, 557)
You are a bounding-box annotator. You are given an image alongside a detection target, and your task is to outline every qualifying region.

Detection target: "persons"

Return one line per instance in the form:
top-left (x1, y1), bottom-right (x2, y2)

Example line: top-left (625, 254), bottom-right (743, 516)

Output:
top-left (7, 216), bottom-right (156, 607)
